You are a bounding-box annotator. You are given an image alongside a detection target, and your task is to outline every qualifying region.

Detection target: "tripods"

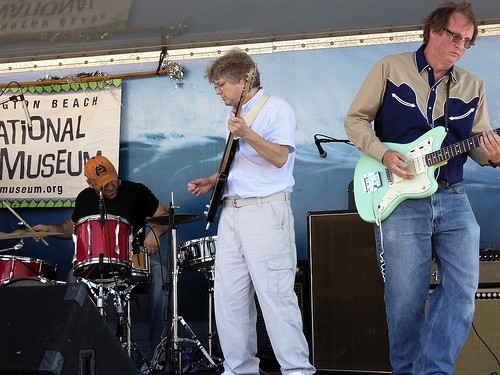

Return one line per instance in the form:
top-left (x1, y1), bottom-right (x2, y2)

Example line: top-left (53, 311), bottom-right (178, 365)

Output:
top-left (121, 220), bottom-right (222, 375)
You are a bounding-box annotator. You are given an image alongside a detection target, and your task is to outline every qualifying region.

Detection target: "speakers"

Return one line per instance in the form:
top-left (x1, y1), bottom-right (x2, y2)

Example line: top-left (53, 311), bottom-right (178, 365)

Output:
top-left (424, 248), bottom-right (500, 375)
top-left (0, 282), bottom-right (142, 375)
top-left (307, 210), bottom-right (393, 375)
top-left (250, 273), bottom-right (306, 375)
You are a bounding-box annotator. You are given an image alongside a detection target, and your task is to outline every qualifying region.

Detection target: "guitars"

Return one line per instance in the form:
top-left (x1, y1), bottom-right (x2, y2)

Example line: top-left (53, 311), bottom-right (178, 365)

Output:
top-left (204, 62), bottom-right (257, 222)
top-left (353, 126), bottom-right (500, 223)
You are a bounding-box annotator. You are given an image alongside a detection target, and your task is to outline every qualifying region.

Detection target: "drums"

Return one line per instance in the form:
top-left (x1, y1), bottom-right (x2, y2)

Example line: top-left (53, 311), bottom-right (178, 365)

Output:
top-left (0, 254), bottom-right (57, 287)
top-left (4, 278), bottom-right (54, 286)
top-left (73, 214), bottom-right (132, 283)
top-left (129, 246), bottom-right (151, 282)
top-left (178, 235), bottom-right (217, 268)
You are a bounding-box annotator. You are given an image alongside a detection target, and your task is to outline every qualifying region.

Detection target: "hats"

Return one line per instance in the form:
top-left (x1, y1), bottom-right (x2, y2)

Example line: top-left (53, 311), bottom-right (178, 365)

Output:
top-left (84, 155), bottom-right (118, 187)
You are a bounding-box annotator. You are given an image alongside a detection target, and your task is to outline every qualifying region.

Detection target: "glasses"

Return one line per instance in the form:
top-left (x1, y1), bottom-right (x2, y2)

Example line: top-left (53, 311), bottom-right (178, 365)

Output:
top-left (214, 79), bottom-right (227, 89)
top-left (443, 27), bottom-right (475, 49)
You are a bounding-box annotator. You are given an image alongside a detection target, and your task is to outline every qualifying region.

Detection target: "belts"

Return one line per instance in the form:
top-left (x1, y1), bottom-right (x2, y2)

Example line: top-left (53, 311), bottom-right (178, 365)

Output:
top-left (222, 190), bottom-right (290, 207)
top-left (437, 179), bottom-right (449, 188)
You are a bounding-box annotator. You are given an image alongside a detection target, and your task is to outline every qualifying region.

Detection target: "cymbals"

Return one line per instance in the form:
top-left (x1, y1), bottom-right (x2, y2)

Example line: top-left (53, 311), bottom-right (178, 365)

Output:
top-left (145, 213), bottom-right (200, 225)
top-left (0, 232), bottom-right (7, 234)
top-left (0, 228), bottom-right (64, 240)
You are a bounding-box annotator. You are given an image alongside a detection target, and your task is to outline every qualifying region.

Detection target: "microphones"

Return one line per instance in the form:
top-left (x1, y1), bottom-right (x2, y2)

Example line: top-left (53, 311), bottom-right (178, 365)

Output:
top-left (98, 188), bottom-right (105, 213)
top-left (314, 135), bottom-right (327, 158)
top-left (133, 226), bottom-right (146, 239)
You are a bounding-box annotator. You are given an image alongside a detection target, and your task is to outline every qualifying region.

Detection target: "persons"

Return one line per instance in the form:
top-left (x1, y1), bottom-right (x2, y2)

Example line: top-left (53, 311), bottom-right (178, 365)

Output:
top-left (29, 155), bottom-right (169, 375)
top-left (344, 2), bottom-right (500, 375)
top-left (187, 50), bottom-right (316, 375)
